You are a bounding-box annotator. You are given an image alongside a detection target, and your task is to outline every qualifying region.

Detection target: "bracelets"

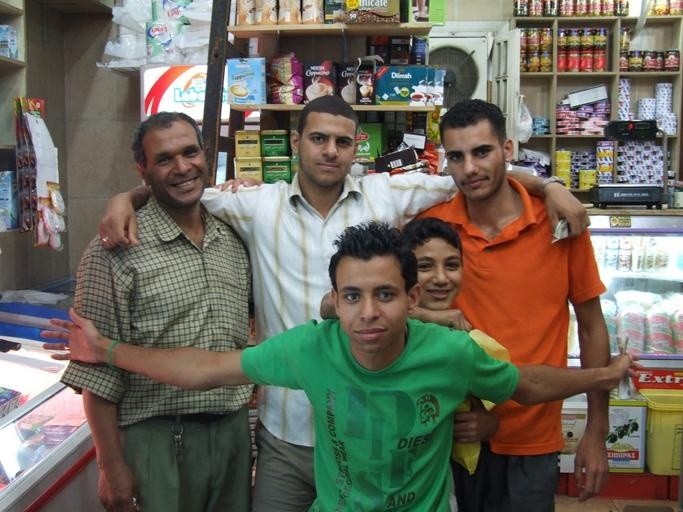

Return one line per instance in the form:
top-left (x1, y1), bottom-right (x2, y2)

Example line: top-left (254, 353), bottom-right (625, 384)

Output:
top-left (536, 173), bottom-right (568, 190)
top-left (104, 337), bottom-right (122, 373)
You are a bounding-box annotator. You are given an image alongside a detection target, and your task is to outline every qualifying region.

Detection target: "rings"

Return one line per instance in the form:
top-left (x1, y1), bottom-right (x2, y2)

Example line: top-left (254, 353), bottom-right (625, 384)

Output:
top-left (99, 234), bottom-right (111, 245)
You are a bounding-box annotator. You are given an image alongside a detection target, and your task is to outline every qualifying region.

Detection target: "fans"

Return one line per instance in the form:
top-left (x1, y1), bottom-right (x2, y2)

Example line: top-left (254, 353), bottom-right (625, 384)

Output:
top-left (423, 29), bottom-right (496, 118)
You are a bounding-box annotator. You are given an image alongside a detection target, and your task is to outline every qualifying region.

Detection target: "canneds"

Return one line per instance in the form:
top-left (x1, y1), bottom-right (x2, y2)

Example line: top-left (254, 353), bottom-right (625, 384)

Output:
top-left (514, 0), bottom-right (683, 189)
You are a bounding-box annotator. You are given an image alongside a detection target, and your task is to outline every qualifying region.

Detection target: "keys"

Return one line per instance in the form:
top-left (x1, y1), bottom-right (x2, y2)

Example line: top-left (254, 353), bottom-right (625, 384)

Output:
top-left (171, 431), bottom-right (184, 461)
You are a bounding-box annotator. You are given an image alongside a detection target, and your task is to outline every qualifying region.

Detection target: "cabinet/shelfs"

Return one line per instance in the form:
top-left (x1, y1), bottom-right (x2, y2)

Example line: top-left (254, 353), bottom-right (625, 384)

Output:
top-left (580, 202), bottom-right (683, 295)
top-left (226, 21), bottom-right (435, 186)
top-left (509, 14), bottom-right (683, 205)
top-left (0, 0), bottom-right (29, 238)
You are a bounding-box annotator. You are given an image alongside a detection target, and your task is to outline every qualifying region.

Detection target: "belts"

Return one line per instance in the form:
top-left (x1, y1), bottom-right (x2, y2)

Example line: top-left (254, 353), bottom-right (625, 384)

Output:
top-left (154, 408), bottom-right (233, 425)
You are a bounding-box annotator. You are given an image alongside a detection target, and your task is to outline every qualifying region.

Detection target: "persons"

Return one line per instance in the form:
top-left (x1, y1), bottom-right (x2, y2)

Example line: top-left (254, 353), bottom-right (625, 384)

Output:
top-left (87, 93), bottom-right (586, 511)
top-left (307, 211), bottom-right (512, 512)
top-left (37, 217), bottom-right (644, 511)
top-left (57, 111), bottom-right (264, 511)
top-left (390, 98), bottom-right (621, 510)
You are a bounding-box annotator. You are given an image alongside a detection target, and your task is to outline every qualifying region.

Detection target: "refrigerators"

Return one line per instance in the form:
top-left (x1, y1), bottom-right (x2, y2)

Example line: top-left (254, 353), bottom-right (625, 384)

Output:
top-left (0, 275), bottom-right (89, 347)
top-left (0, 322), bottom-right (106, 511)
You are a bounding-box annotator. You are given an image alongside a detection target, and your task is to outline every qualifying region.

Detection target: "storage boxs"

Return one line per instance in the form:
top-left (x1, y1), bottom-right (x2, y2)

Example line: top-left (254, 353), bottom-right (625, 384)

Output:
top-left (556, 387), bottom-right (683, 477)
